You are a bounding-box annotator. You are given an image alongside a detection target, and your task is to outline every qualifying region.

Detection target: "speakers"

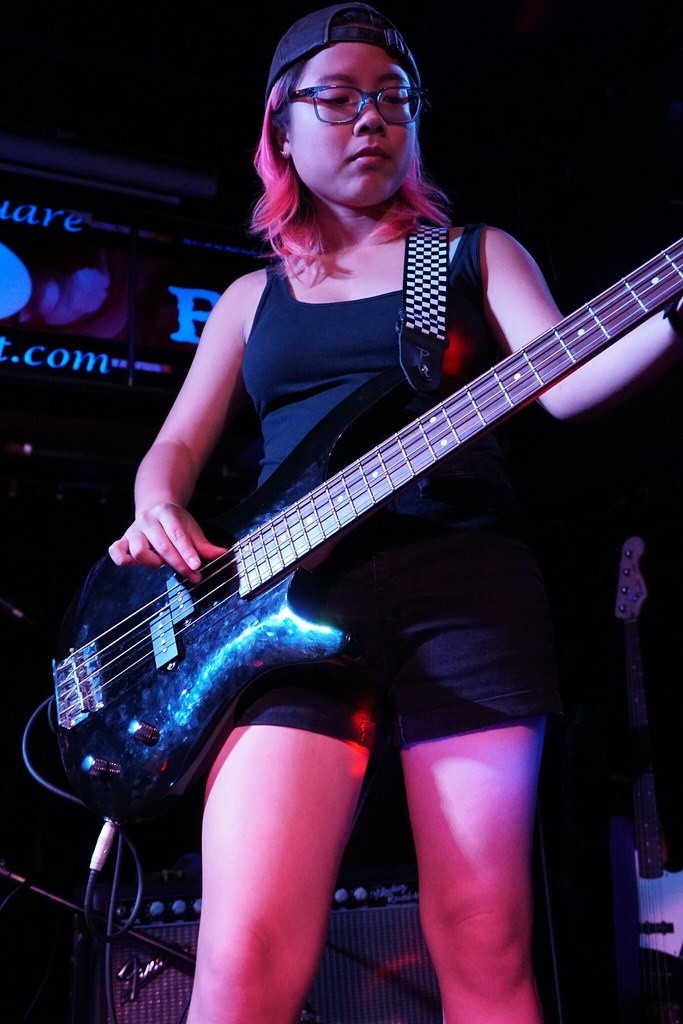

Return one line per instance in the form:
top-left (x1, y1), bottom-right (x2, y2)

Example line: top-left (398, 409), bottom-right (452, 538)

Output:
top-left (93, 847), bottom-right (568, 1024)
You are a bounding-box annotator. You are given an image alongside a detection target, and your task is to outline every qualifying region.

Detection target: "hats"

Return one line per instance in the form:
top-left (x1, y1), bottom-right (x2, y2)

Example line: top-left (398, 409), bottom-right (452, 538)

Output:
top-left (265, 2), bottom-right (420, 106)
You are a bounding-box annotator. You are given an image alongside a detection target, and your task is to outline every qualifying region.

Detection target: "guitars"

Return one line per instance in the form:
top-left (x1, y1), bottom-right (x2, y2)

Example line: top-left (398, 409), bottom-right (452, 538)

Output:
top-left (35, 241), bottom-right (683, 823)
top-left (611, 533), bottom-right (683, 1024)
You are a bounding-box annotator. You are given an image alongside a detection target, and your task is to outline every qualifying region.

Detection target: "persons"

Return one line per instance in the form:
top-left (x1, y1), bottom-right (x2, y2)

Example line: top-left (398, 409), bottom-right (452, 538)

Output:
top-left (109, 5), bottom-right (683, 1024)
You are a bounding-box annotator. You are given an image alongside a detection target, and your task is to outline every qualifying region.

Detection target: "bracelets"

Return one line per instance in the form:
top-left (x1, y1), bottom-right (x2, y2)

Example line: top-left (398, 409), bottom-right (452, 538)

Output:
top-left (663, 302), bottom-right (683, 335)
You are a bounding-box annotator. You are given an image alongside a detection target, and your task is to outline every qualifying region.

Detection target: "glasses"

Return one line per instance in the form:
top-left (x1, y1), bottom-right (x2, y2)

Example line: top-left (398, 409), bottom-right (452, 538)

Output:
top-left (287, 85), bottom-right (424, 125)
